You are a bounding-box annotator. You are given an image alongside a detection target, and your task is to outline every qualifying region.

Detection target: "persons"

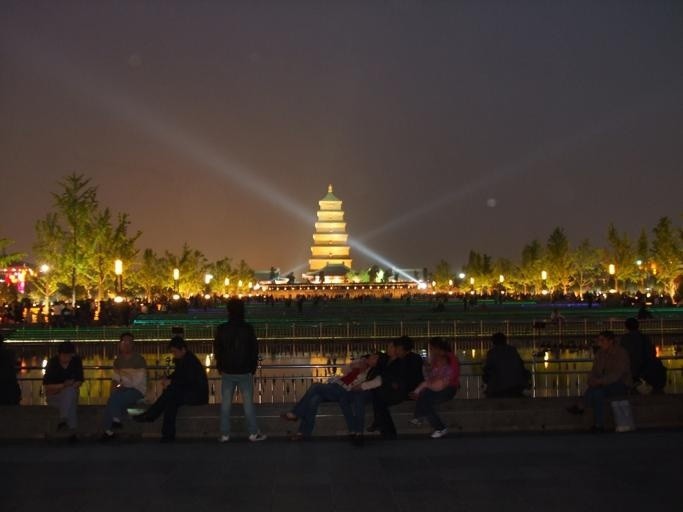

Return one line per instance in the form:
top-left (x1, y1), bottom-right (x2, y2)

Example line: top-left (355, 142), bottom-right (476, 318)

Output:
top-left (481, 334), bottom-right (530, 398)
top-left (104, 332), bottom-right (148, 443)
top-left (582, 331), bottom-right (632, 417)
top-left (213, 299), bottom-right (267, 443)
top-left (42, 341), bottom-right (84, 441)
top-left (280, 336), bottom-right (461, 440)
top-left (132, 335), bottom-right (209, 450)
top-left (617, 317), bottom-right (668, 396)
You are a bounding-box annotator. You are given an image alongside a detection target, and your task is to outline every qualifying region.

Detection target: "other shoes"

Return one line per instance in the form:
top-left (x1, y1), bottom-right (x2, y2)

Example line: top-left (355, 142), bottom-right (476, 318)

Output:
top-left (347, 432), bottom-right (364, 446)
top-left (67, 435), bottom-right (83, 442)
top-left (56, 423), bottom-right (74, 433)
top-left (372, 431), bottom-right (391, 442)
top-left (133, 414), bottom-right (154, 423)
top-left (159, 436), bottom-right (175, 444)
top-left (112, 419), bottom-right (123, 431)
top-left (287, 431), bottom-right (303, 441)
top-left (280, 412), bottom-right (300, 428)
top-left (408, 417), bottom-right (427, 426)
top-left (249, 433), bottom-right (267, 441)
top-left (432, 429), bottom-right (447, 438)
top-left (218, 435), bottom-right (230, 441)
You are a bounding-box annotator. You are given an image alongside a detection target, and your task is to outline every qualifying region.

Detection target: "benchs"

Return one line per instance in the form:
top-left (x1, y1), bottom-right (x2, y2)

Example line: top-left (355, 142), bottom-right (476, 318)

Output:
top-left (1, 393), bottom-right (683, 439)
